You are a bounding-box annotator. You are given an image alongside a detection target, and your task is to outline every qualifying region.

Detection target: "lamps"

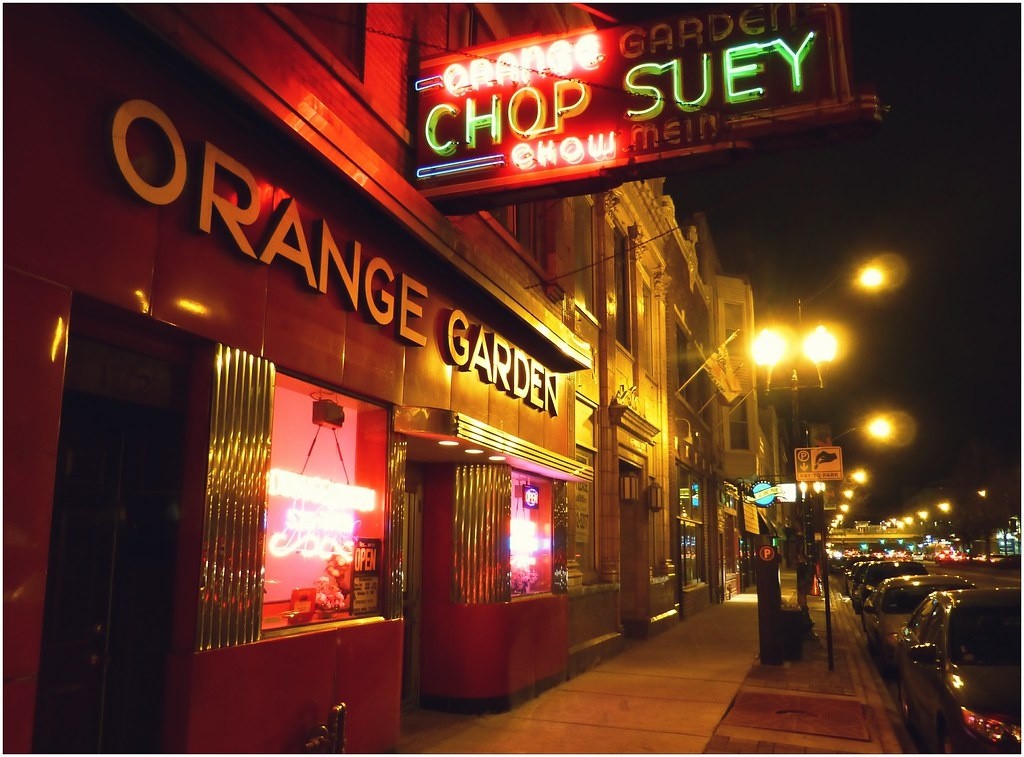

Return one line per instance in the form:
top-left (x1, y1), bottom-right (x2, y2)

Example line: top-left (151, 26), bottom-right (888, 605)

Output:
top-left (674, 417), bottom-right (694, 445)
top-left (648, 475), bottom-right (663, 513)
top-left (619, 468), bottom-right (639, 504)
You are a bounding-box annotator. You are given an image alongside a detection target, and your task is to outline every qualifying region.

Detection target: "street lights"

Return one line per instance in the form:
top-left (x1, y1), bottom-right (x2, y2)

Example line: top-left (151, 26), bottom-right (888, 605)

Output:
top-left (748, 321), bottom-right (834, 675)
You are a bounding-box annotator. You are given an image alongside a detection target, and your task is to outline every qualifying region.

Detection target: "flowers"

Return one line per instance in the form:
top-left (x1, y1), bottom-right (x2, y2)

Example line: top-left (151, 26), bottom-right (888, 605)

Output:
top-left (313, 573), bottom-right (344, 611)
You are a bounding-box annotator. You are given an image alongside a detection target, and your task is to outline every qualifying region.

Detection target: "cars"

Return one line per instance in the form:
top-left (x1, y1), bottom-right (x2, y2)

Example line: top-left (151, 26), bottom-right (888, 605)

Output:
top-left (863, 573), bottom-right (977, 667)
top-left (893, 586), bottom-right (1022, 754)
top-left (834, 550), bottom-right (930, 615)
